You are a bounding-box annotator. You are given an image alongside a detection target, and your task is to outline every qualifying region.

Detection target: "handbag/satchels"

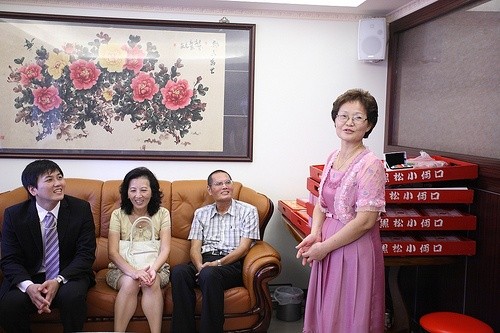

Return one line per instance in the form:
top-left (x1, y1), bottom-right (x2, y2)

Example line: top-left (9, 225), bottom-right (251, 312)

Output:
top-left (119, 217), bottom-right (161, 271)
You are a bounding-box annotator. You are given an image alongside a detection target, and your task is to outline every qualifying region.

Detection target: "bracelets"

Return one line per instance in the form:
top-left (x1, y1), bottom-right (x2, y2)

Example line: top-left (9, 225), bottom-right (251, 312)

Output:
top-left (217, 260), bottom-right (222, 267)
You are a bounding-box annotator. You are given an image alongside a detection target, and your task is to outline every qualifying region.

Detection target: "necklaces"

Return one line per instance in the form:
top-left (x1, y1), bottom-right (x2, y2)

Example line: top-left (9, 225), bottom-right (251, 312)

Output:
top-left (133, 211), bottom-right (147, 228)
top-left (40, 223), bottom-right (57, 230)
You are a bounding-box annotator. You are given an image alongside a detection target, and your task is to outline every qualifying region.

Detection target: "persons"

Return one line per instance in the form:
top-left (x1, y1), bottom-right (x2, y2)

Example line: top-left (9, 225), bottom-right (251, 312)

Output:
top-left (105, 167), bottom-right (171, 333)
top-left (295, 88), bottom-right (385, 333)
top-left (170, 170), bottom-right (260, 333)
top-left (0, 160), bottom-right (96, 333)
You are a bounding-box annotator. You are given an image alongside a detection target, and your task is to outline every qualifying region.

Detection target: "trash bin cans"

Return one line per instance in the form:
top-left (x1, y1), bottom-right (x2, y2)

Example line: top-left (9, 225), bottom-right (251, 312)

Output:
top-left (273, 285), bottom-right (304, 322)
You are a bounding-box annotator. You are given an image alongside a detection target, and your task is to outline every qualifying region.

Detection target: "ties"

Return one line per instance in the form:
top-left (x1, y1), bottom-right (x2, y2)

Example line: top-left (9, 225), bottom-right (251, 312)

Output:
top-left (45, 213), bottom-right (59, 281)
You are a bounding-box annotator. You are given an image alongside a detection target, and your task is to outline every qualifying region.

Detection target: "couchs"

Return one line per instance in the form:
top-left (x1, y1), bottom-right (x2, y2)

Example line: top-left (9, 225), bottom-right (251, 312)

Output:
top-left (0, 178), bottom-right (281, 333)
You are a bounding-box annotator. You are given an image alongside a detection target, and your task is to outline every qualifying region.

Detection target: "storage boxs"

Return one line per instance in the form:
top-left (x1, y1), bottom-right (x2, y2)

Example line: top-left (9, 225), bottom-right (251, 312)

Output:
top-left (278, 154), bottom-right (479, 256)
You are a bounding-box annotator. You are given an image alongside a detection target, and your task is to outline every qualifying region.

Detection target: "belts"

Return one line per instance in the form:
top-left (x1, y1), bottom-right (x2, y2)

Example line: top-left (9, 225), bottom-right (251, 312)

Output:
top-left (202, 251), bottom-right (228, 256)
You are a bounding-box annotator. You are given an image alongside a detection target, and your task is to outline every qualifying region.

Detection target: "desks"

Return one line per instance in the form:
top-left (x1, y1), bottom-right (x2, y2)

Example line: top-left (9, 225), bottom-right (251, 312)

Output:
top-left (281, 213), bottom-right (465, 333)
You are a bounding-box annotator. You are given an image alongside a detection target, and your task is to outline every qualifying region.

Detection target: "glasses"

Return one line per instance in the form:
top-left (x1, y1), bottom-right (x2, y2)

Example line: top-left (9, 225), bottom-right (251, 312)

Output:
top-left (336, 114), bottom-right (368, 124)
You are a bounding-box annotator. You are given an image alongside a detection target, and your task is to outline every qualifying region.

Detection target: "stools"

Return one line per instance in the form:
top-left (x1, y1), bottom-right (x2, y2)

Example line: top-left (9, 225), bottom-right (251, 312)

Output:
top-left (420, 312), bottom-right (494, 333)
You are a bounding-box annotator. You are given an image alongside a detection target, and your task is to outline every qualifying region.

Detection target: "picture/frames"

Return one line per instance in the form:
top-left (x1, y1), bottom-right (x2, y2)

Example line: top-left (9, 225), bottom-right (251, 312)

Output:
top-left (0, 11), bottom-right (256, 162)
